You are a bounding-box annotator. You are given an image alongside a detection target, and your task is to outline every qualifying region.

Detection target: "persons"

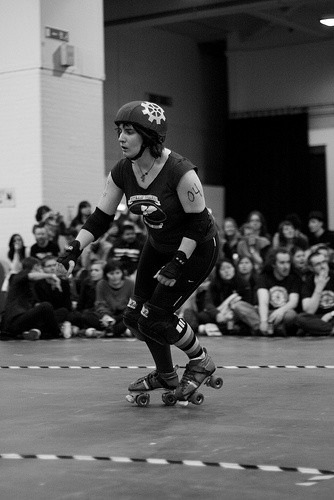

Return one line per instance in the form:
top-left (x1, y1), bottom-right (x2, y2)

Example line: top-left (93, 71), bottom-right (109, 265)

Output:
top-left (0, 201), bottom-right (334, 340)
top-left (56, 100), bottom-right (223, 406)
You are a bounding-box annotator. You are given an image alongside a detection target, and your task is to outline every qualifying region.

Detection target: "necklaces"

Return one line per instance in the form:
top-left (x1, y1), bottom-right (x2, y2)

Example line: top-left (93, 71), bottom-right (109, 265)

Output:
top-left (139, 159), bottom-right (156, 182)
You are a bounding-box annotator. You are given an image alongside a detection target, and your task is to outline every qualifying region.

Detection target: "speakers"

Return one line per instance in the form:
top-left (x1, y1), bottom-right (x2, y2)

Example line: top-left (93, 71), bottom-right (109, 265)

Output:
top-left (60, 43), bottom-right (74, 66)
